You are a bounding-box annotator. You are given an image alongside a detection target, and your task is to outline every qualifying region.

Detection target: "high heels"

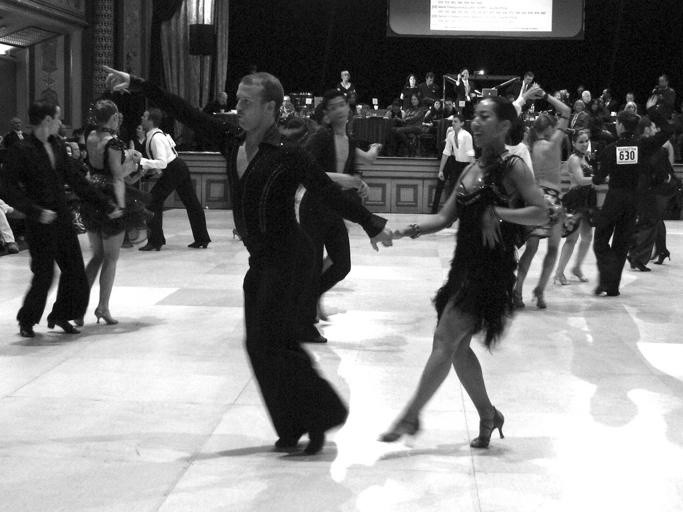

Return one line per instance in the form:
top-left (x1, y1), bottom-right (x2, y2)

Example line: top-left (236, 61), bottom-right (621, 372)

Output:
top-left (470, 406), bottom-right (505, 448)
top-left (510, 288), bottom-right (526, 309)
top-left (138, 242), bottom-right (161, 251)
top-left (47, 311), bottom-right (81, 333)
top-left (95, 306), bottom-right (120, 327)
top-left (654, 248), bottom-right (671, 264)
top-left (187, 240), bottom-right (211, 249)
top-left (377, 414), bottom-right (419, 447)
top-left (532, 285), bottom-right (549, 308)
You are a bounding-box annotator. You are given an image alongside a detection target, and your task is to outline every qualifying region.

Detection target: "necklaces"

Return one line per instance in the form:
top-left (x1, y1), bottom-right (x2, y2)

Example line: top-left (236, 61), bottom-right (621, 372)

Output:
top-left (245, 138), bottom-right (259, 146)
top-left (478, 150), bottom-right (508, 169)
top-left (96, 127), bottom-right (115, 134)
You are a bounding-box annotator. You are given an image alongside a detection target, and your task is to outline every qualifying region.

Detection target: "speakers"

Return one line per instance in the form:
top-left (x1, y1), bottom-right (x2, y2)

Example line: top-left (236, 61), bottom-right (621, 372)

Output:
top-left (189, 24), bottom-right (216, 56)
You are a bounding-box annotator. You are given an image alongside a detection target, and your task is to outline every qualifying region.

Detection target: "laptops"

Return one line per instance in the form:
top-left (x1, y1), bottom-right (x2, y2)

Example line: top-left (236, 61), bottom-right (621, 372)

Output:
top-left (482, 88), bottom-right (497, 97)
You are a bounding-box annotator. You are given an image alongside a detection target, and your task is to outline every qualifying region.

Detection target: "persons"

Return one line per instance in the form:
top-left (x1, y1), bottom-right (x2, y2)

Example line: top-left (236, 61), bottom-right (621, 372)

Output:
top-left (0, 100), bottom-right (119, 337)
top-left (0, 199), bottom-right (26, 255)
top-left (303, 88), bottom-right (369, 343)
top-left (438, 114), bottom-right (475, 197)
top-left (337, 70), bottom-right (356, 97)
top-left (59, 128), bottom-right (90, 234)
top-left (280, 96), bottom-right (295, 115)
top-left (203, 92), bottom-right (231, 116)
top-left (74, 92), bottom-right (143, 327)
top-left (508, 69), bottom-right (681, 307)
top-left (378, 96), bottom-right (549, 451)
top-left (4, 117), bottom-right (28, 147)
top-left (386, 69), bottom-right (479, 159)
top-left (98, 63), bottom-right (397, 456)
top-left (121, 108), bottom-right (210, 251)
top-left (315, 104), bottom-right (383, 322)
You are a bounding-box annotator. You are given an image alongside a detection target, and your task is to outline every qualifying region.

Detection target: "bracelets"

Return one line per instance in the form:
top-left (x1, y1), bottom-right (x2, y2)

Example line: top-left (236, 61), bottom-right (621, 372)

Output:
top-left (119, 207), bottom-right (127, 210)
top-left (407, 223), bottom-right (420, 241)
top-left (544, 92), bottom-right (549, 100)
top-left (487, 204), bottom-right (503, 224)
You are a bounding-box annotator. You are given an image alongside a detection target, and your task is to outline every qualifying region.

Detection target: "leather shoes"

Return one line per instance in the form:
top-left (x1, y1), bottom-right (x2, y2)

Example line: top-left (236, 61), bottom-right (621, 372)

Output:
top-left (16, 317), bottom-right (36, 338)
top-left (303, 333), bottom-right (329, 343)
top-left (275, 432), bottom-right (304, 451)
top-left (307, 410), bottom-right (347, 457)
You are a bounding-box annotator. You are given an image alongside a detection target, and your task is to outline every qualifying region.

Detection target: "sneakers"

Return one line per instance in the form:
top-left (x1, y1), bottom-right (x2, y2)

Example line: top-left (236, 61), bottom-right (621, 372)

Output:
top-left (606, 288), bottom-right (622, 296)
top-left (571, 266), bottom-right (591, 283)
top-left (553, 269), bottom-right (570, 285)
top-left (595, 287), bottom-right (604, 295)
top-left (74, 315), bottom-right (85, 326)
top-left (630, 259), bottom-right (652, 272)
